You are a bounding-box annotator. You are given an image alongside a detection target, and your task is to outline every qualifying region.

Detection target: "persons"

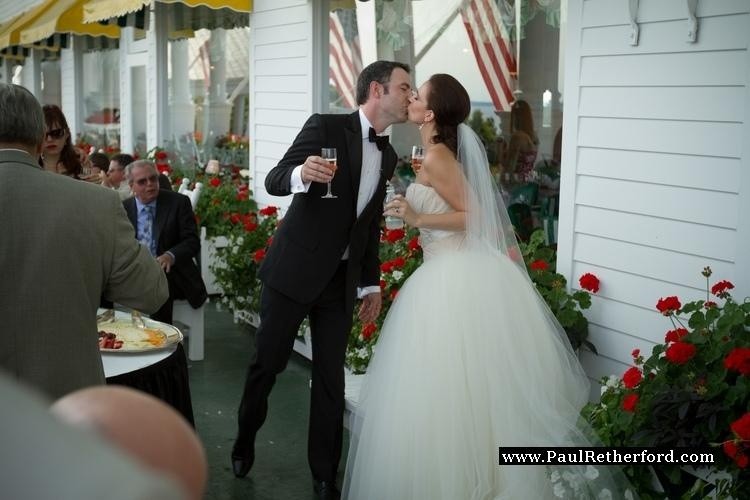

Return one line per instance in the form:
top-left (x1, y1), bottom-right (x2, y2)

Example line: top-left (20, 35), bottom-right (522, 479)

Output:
top-left (38, 103), bottom-right (94, 182)
top-left (100, 159), bottom-right (208, 326)
top-left (1, 83), bottom-right (170, 403)
top-left (87, 152), bottom-right (109, 175)
top-left (501, 100), bottom-right (539, 186)
top-left (231, 60), bottom-right (413, 500)
top-left (99, 154), bottom-right (136, 203)
top-left (341, 74), bottom-right (637, 500)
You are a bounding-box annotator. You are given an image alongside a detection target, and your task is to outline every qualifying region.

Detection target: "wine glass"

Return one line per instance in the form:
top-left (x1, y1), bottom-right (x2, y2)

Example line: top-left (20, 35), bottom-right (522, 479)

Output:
top-left (321, 147), bottom-right (337, 199)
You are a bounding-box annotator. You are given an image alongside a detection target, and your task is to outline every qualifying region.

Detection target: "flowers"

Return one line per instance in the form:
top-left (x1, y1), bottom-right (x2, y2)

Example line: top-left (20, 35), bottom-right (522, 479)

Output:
top-left (75, 141), bottom-right (121, 161)
top-left (189, 164), bottom-right (253, 240)
top-left (132, 146), bottom-right (182, 186)
top-left (345, 225), bottom-right (422, 373)
top-left (582, 265), bottom-right (750, 500)
top-left (507, 228), bottom-right (600, 356)
top-left (208, 206), bottom-right (284, 313)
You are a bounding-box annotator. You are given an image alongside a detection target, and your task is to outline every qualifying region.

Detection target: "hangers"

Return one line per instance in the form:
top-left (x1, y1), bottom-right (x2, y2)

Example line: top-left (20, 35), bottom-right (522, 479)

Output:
top-left (96, 307), bottom-right (196, 431)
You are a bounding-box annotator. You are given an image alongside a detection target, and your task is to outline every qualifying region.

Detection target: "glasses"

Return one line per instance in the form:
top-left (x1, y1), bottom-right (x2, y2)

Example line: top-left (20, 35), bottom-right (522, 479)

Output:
top-left (137, 176), bottom-right (156, 186)
top-left (109, 168), bottom-right (121, 172)
top-left (46, 129), bottom-right (64, 140)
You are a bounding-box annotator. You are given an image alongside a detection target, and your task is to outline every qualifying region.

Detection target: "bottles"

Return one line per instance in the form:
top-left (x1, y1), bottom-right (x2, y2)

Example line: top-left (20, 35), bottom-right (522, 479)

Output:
top-left (383, 186), bottom-right (404, 230)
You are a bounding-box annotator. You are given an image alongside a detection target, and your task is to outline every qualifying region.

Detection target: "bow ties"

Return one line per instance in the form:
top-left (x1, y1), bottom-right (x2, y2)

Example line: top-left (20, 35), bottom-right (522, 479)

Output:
top-left (369, 128), bottom-right (389, 151)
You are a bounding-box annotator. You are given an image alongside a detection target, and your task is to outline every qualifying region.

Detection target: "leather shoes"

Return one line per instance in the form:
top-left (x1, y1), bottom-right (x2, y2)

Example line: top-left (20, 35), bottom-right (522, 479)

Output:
top-left (232, 453), bottom-right (254, 477)
top-left (313, 480), bottom-right (341, 500)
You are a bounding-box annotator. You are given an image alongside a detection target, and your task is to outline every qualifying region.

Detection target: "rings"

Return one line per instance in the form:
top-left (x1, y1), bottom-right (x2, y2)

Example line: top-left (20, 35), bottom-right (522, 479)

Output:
top-left (394, 209), bottom-right (400, 214)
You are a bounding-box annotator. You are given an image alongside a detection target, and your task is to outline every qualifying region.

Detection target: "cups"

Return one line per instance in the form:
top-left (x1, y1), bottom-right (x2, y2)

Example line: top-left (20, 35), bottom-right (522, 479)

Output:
top-left (410, 145), bottom-right (423, 173)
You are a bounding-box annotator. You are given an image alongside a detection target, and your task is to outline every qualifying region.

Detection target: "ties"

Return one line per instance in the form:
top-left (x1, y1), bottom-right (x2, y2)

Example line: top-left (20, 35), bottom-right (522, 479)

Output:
top-left (142, 207), bottom-right (153, 252)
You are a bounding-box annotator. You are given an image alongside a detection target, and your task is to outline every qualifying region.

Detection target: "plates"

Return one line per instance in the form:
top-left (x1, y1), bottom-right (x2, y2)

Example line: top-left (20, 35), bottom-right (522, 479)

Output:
top-left (95, 320), bottom-right (183, 355)
